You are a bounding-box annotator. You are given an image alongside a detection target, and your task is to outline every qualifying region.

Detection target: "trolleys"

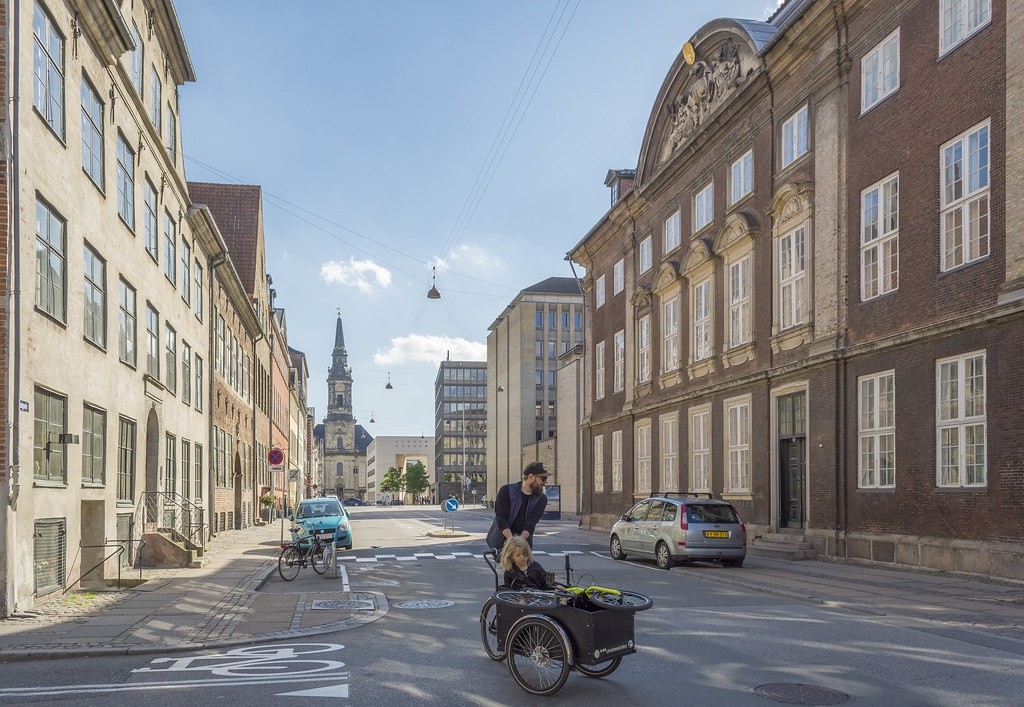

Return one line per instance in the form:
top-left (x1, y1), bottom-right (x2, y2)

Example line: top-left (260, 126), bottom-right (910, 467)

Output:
top-left (479, 546), bottom-right (638, 696)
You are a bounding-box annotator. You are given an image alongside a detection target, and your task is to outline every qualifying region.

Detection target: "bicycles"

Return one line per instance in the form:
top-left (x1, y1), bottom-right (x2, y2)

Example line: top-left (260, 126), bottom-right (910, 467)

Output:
top-left (277, 519), bottom-right (333, 582)
top-left (488, 553), bottom-right (656, 616)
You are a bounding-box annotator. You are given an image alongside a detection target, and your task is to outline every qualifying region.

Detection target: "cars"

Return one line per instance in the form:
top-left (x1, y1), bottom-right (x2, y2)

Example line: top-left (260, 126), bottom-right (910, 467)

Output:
top-left (285, 496), bottom-right (354, 553)
top-left (341, 497), bottom-right (362, 507)
top-left (607, 491), bottom-right (747, 569)
top-left (325, 494), bottom-right (341, 502)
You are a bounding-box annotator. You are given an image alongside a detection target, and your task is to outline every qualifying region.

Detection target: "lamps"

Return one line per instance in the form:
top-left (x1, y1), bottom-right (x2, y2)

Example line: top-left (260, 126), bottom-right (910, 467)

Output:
top-left (386, 372), bottom-right (395, 390)
top-left (370, 412), bottom-right (375, 423)
top-left (427, 266), bottom-right (440, 299)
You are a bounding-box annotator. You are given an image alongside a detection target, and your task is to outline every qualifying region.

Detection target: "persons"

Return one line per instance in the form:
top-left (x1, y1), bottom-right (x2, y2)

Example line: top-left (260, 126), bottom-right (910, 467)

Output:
top-left (449, 493), bottom-right (458, 501)
top-left (486, 461), bottom-right (552, 550)
top-left (501, 535), bottom-right (548, 592)
top-left (422, 496), bottom-right (430, 504)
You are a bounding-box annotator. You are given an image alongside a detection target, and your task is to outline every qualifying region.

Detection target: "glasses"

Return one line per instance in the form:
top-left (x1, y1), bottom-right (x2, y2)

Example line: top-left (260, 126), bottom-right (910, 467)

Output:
top-left (535, 475), bottom-right (547, 482)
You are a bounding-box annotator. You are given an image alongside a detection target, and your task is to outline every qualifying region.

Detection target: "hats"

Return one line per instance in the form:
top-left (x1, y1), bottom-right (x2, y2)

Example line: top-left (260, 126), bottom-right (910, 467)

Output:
top-left (523, 462), bottom-right (551, 476)
